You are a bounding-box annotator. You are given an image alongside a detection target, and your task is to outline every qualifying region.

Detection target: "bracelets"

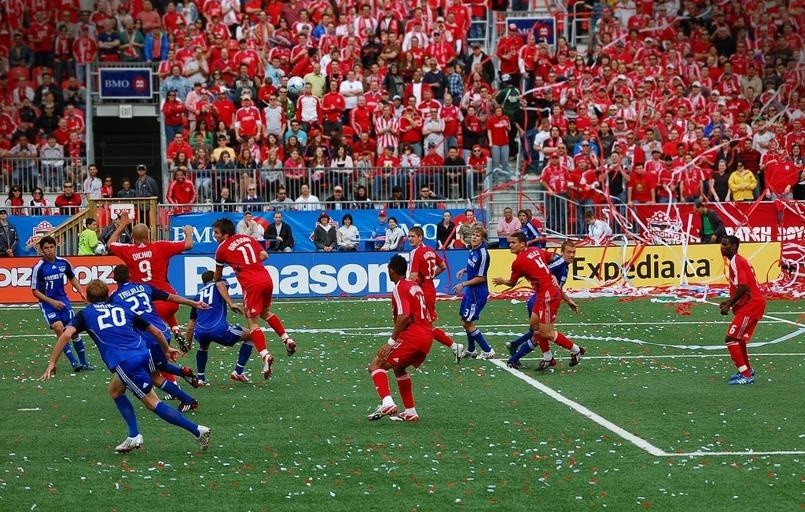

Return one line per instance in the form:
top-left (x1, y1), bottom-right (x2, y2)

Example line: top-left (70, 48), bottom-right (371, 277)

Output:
top-left (462, 282), bottom-right (464, 288)
top-left (386, 337), bottom-right (396, 347)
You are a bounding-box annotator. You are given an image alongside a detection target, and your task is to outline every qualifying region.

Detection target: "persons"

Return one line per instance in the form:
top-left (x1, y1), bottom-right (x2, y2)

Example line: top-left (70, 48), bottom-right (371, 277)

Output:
top-left (456, 226), bottom-right (496, 360)
top-left (106, 212), bottom-right (194, 401)
top-left (108, 265), bottom-right (213, 414)
top-left (211, 219), bottom-right (296, 380)
top-left (720, 235), bottom-right (767, 385)
top-left (39, 279), bottom-right (211, 453)
top-left (367, 256), bottom-right (435, 422)
top-left (1, 0), bottom-right (805, 257)
top-left (492, 232), bottom-right (587, 371)
top-left (504, 241), bottom-right (580, 369)
top-left (408, 227), bottom-right (464, 364)
top-left (29, 236), bottom-right (95, 372)
top-left (180, 270), bottom-right (255, 387)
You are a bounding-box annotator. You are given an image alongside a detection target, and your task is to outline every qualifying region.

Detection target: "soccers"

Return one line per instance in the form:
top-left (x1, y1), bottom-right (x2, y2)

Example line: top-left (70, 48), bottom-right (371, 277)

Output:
top-left (286, 76), bottom-right (307, 95)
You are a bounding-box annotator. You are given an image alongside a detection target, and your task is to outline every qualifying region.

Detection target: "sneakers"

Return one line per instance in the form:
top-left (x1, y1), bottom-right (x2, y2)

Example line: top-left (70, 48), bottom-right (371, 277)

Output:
top-left (73, 331), bottom-right (297, 455)
top-left (728, 376), bottom-right (754, 385)
top-left (731, 368), bottom-right (755, 379)
top-left (368, 341), bottom-right (586, 422)
